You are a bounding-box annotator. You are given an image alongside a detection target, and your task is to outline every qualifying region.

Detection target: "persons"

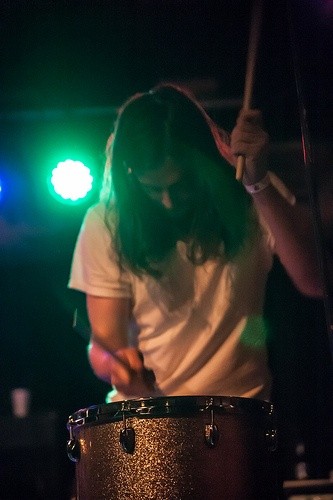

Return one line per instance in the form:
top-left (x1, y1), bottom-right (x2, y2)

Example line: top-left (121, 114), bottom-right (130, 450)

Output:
top-left (65, 84), bottom-right (325, 409)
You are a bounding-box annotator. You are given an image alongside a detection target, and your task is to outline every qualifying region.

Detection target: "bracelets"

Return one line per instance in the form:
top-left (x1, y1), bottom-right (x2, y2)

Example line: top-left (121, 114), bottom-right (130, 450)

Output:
top-left (242, 173), bottom-right (272, 193)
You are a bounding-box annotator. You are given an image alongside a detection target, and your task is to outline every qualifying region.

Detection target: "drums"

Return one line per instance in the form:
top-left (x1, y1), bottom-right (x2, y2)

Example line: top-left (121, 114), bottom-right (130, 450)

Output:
top-left (66, 395), bottom-right (289, 500)
